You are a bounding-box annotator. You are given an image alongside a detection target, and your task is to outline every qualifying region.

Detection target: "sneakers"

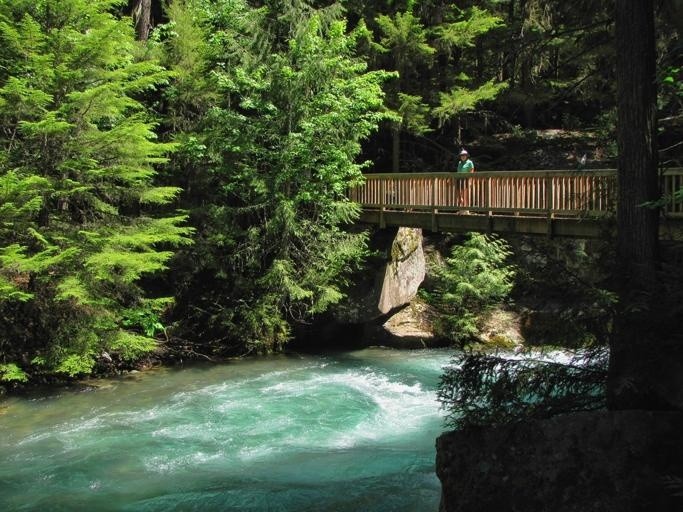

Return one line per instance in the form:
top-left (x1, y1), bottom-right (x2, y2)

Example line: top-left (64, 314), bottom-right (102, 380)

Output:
top-left (457, 209), bottom-right (470, 215)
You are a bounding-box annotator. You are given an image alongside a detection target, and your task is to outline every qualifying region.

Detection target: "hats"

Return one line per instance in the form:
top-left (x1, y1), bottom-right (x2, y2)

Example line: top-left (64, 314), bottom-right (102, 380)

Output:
top-left (460, 150), bottom-right (468, 155)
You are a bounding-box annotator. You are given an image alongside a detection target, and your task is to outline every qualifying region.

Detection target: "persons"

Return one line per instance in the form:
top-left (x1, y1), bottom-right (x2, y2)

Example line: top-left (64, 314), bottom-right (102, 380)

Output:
top-left (455, 149), bottom-right (475, 216)
